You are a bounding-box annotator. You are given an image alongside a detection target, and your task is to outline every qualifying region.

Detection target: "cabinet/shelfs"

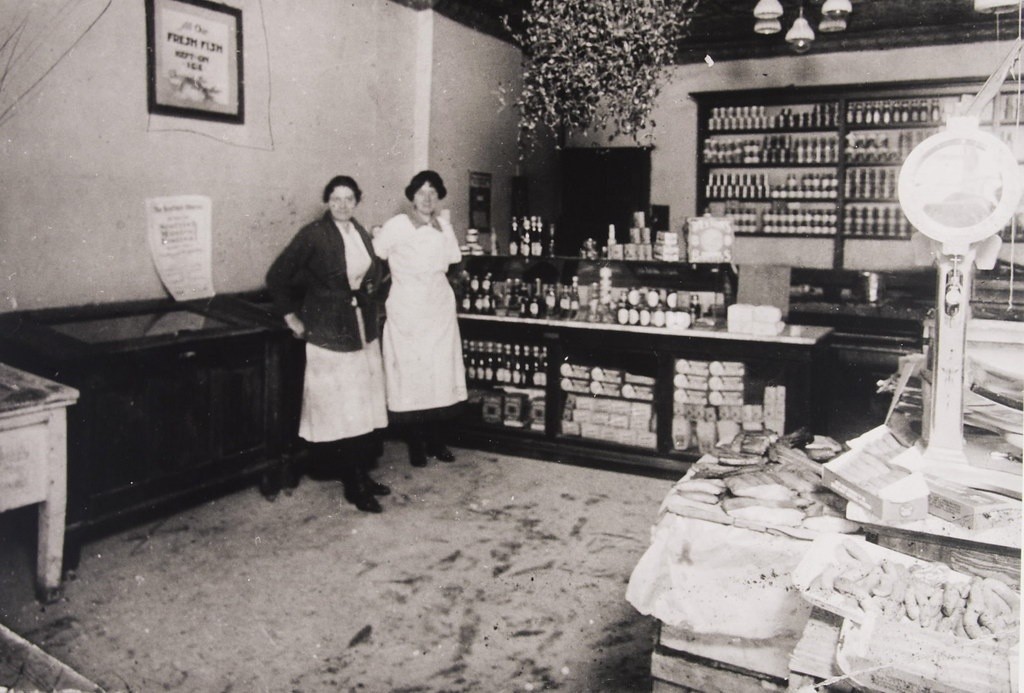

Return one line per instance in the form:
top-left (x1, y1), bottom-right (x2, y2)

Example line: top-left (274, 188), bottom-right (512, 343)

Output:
top-left (0, 286), bottom-right (302, 582)
top-left (689, 72), bottom-right (1024, 243)
top-left (443, 254), bottom-right (935, 481)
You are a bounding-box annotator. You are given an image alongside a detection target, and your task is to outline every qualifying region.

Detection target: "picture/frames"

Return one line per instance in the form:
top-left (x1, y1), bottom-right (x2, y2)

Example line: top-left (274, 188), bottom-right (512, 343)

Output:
top-left (144, 0), bottom-right (245, 125)
top-left (468, 170), bottom-right (492, 234)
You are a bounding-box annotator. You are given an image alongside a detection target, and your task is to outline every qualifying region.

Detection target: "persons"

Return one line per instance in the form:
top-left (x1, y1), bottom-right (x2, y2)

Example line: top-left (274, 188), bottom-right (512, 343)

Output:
top-left (372, 170), bottom-right (468, 467)
top-left (265, 175), bottom-right (392, 512)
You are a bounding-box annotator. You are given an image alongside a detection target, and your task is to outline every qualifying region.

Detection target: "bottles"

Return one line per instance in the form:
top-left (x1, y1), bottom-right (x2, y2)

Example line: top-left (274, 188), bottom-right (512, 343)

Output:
top-left (458, 270), bottom-right (701, 329)
top-left (508, 215), bottom-right (556, 257)
top-left (461, 339), bottom-right (547, 386)
top-left (705, 99), bottom-right (939, 234)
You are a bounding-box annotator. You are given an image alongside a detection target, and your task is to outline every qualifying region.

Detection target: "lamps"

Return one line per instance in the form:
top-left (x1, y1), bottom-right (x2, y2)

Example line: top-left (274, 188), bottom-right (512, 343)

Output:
top-left (785, 6), bottom-right (815, 53)
top-left (753, 0), bottom-right (784, 20)
top-left (821, 0), bottom-right (853, 17)
top-left (754, 20), bottom-right (781, 34)
top-left (818, 18), bottom-right (847, 33)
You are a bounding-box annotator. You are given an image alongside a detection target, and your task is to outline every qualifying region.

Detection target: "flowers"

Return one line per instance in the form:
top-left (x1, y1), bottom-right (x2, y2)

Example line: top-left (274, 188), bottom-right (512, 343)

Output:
top-left (496, 0), bottom-right (699, 163)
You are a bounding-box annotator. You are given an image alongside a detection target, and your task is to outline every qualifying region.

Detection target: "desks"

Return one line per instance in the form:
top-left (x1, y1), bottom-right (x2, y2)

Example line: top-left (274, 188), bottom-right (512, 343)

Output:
top-left (0, 362), bottom-right (80, 607)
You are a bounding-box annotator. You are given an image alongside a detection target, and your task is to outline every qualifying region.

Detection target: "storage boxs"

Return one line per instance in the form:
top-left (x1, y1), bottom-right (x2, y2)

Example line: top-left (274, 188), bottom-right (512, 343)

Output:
top-left (561, 396), bottom-right (657, 449)
top-left (821, 425), bottom-right (1019, 532)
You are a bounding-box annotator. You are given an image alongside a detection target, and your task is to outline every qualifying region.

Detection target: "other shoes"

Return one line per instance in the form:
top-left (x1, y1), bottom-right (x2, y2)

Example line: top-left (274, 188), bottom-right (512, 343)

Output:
top-left (345, 487), bottom-right (383, 513)
top-left (427, 441), bottom-right (454, 462)
top-left (360, 475), bottom-right (390, 495)
top-left (410, 446), bottom-right (427, 467)
